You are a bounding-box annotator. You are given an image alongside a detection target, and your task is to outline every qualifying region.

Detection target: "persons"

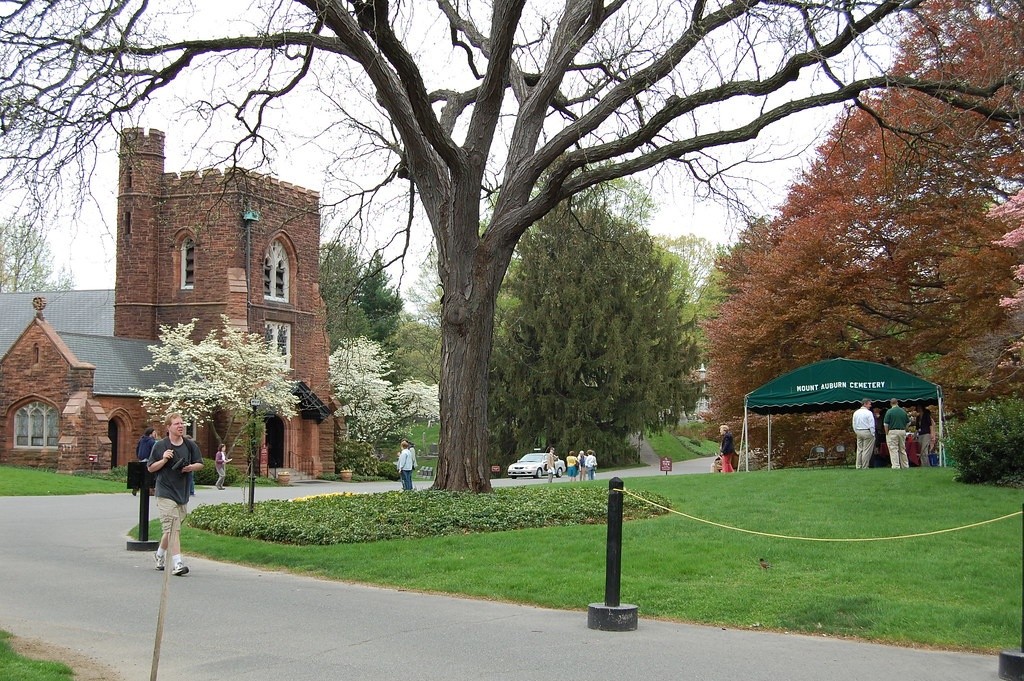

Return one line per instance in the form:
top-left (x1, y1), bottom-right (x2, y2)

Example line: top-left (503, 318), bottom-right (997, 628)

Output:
top-left (397, 437), bottom-right (418, 490)
top-left (853, 398), bottom-right (887, 469)
top-left (215, 444), bottom-right (232, 490)
top-left (914, 403), bottom-right (935, 467)
top-left (884, 398), bottom-right (910, 469)
top-left (566, 450), bottom-right (597, 482)
top-left (719, 425), bottom-right (734, 473)
top-left (132, 412), bottom-right (205, 576)
top-left (547, 447), bottom-right (557, 484)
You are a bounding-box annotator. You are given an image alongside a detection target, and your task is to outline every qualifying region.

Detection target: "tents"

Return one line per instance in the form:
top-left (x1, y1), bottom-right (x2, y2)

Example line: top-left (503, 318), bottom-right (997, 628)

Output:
top-left (744, 357), bottom-right (946, 471)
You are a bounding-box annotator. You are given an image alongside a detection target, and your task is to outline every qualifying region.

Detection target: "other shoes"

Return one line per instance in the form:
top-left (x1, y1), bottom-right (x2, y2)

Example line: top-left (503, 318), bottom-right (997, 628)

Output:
top-left (132, 489), bottom-right (137, 496)
top-left (190, 494), bottom-right (196, 496)
top-left (149, 494), bottom-right (154, 496)
top-left (215, 485), bottom-right (225, 490)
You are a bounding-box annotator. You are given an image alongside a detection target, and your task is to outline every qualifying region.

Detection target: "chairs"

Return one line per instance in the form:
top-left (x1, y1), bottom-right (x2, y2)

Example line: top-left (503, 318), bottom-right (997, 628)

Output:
top-left (916, 440), bottom-right (929, 467)
top-left (806, 445), bottom-right (826, 471)
top-left (826, 443), bottom-right (848, 469)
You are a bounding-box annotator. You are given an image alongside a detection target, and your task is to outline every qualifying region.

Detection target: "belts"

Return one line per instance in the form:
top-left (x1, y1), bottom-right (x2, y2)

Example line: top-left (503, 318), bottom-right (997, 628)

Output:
top-left (889, 428), bottom-right (904, 430)
top-left (857, 429), bottom-right (870, 430)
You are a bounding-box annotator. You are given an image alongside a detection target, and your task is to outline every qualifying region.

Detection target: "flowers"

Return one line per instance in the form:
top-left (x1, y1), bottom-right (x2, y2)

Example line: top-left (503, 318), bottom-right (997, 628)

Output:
top-left (342, 468), bottom-right (352, 472)
top-left (278, 471), bottom-right (290, 475)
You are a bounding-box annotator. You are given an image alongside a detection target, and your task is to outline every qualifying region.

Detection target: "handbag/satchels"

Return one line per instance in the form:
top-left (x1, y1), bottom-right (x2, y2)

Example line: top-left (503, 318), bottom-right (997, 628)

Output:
top-left (548, 468), bottom-right (557, 474)
top-left (575, 461), bottom-right (579, 470)
top-left (579, 465), bottom-right (582, 472)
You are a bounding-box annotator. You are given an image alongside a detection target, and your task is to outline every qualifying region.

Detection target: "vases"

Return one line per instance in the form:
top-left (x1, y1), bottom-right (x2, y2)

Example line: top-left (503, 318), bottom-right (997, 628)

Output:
top-left (278, 475), bottom-right (290, 486)
top-left (340, 471), bottom-right (353, 482)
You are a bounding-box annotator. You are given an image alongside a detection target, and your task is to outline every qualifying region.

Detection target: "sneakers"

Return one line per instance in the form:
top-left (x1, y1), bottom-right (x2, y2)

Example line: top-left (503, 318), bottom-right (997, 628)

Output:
top-left (152, 552), bottom-right (165, 570)
top-left (172, 562), bottom-right (189, 576)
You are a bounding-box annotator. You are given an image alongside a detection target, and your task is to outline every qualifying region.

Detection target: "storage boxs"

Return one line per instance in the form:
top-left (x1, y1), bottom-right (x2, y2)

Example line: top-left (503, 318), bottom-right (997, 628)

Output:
top-left (929, 453), bottom-right (938, 466)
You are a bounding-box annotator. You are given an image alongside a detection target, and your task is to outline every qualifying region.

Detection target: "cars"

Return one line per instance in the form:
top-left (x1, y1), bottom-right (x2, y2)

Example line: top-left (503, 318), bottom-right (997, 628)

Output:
top-left (508, 453), bottom-right (567, 479)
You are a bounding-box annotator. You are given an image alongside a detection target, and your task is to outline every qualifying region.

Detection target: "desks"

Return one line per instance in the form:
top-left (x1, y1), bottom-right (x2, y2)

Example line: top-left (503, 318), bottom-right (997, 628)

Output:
top-left (880, 441), bottom-right (922, 466)
